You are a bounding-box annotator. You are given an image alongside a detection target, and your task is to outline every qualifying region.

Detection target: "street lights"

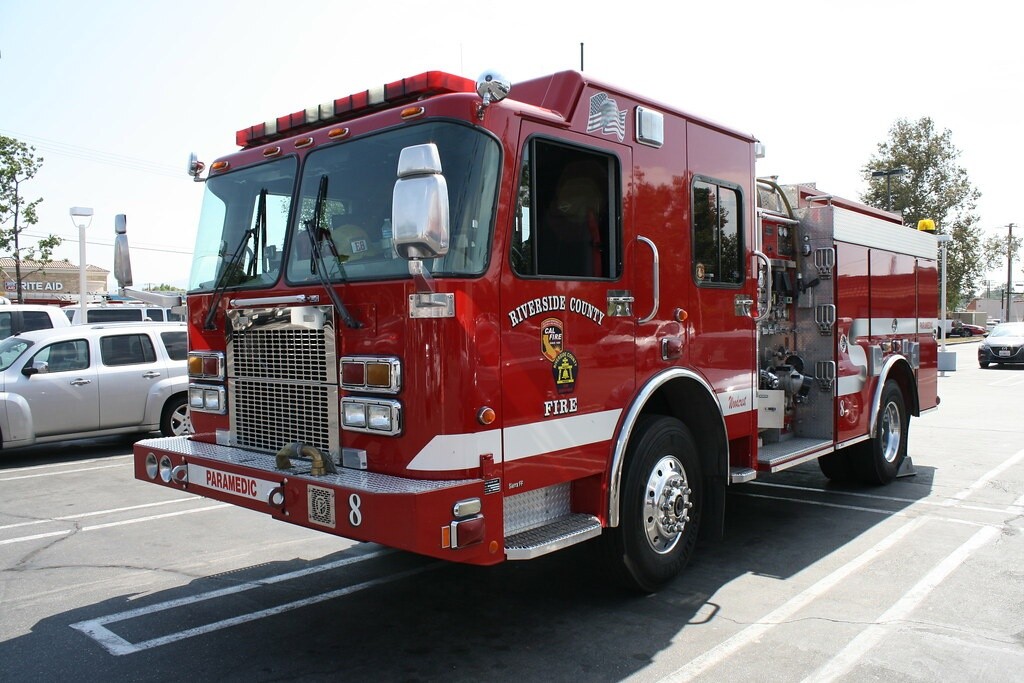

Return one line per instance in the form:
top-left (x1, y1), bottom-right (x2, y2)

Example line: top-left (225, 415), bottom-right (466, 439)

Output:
top-left (70, 206), bottom-right (96, 324)
top-left (872, 169), bottom-right (907, 213)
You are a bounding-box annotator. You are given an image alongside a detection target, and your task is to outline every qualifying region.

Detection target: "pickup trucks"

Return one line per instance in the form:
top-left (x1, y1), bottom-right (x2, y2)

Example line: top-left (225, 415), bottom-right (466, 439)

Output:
top-left (937, 319), bottom-right (962, 338)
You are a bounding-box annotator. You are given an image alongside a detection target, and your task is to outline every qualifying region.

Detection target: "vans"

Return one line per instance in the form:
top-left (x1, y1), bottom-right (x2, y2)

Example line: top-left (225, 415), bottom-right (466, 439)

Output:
top-left (0, 296), bottom-right (187, 369)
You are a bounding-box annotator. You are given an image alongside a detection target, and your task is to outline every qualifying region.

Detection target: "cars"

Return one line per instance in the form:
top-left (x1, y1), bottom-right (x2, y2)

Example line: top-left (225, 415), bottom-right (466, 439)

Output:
top-left (960, 322), bottom-right (985, 338)
top-left (977, 321), bottom-right (1024, 368)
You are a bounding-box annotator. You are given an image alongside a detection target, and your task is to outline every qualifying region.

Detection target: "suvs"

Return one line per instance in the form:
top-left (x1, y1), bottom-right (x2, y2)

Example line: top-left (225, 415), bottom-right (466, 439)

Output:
top-left (0, 319), bottom-right (191, 452)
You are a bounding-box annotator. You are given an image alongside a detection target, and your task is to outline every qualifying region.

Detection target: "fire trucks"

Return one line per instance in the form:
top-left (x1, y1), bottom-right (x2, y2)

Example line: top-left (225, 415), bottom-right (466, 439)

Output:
top-left (113, 41), bottom-right (958, 598)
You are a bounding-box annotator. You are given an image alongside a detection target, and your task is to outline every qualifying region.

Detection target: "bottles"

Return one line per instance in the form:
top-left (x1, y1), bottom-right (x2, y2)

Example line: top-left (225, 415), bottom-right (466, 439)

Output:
top-left (381, 218), bottom-right (392, 259)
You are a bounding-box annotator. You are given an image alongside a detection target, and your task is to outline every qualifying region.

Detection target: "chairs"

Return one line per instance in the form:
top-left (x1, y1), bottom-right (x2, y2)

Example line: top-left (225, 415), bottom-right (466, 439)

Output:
top-left (54, 343), bottom-right (81, 373)
top-left (131, 342), bottom-right (148, 363)
top-left (537, 166), bottom-right (604, 276)
